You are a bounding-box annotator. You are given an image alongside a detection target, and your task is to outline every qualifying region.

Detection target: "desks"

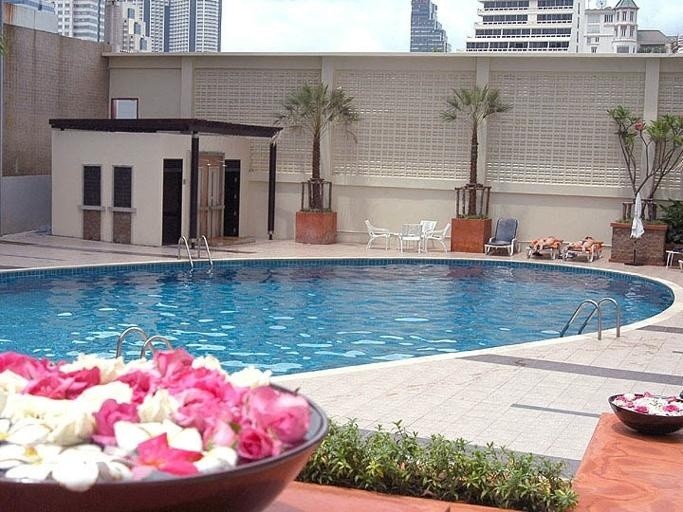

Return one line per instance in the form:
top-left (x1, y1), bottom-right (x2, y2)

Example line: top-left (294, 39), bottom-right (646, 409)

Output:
top-left (666, 250), bottom-right (683, 270)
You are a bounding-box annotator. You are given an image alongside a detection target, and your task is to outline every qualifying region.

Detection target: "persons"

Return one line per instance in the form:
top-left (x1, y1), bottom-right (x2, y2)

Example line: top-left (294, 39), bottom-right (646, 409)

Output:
top-left (572, 235), bottom-right (603, 252)
top-left (533, 236), bottom-right (554, 249)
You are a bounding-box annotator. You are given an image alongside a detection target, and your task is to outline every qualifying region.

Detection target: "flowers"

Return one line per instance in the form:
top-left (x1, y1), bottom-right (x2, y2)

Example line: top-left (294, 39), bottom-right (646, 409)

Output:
top-left (613, 392), bottom-right (683, 417)
top-left (0, 348), bottom-right (312, 493)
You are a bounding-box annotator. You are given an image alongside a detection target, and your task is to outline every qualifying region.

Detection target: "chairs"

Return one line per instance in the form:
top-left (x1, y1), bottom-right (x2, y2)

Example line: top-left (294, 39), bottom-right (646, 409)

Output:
top-left (365, 218), bottom-right (603, 263)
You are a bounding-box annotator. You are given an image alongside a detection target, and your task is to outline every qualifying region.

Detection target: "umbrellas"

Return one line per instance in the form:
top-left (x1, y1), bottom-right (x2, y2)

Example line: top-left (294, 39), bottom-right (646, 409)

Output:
top-left (628, 192), bottom-right (644, 263)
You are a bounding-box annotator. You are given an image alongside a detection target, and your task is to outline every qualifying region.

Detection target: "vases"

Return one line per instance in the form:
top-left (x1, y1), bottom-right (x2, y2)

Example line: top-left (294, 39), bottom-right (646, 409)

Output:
top-left (608, 394), bottom-right (683, 434)
top-left (0, 385), bottom-right (328, 512)
